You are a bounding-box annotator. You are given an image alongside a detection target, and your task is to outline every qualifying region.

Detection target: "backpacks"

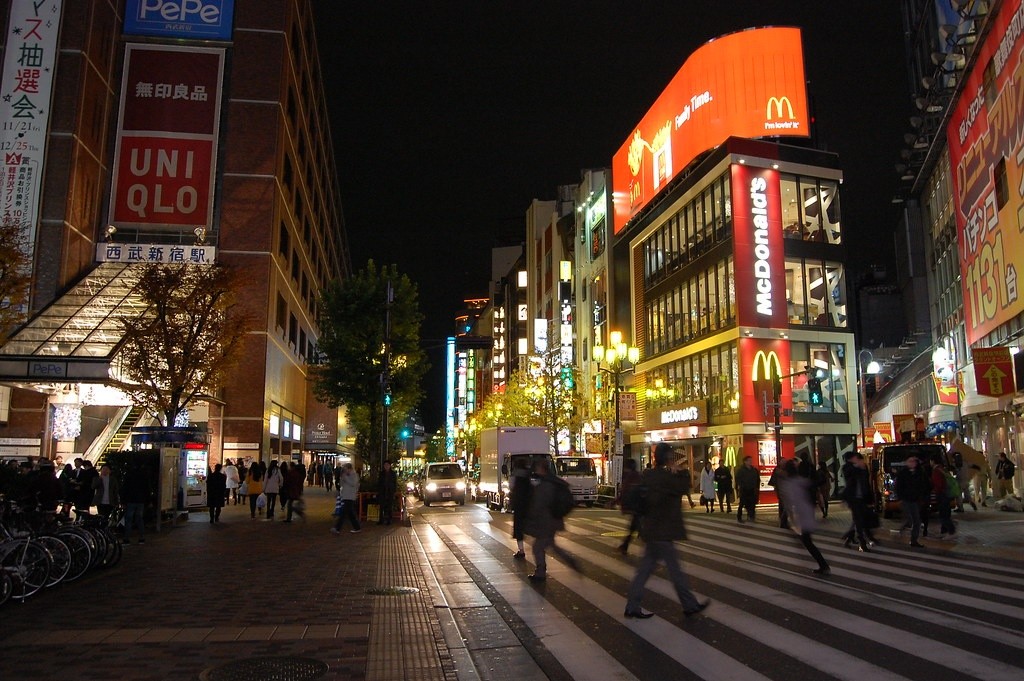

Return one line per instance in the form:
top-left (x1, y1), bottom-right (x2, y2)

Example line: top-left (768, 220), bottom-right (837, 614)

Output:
top-left (549, 487), bottom-right (575, 519)
top-left (937, 468), bottom-right (961, 499)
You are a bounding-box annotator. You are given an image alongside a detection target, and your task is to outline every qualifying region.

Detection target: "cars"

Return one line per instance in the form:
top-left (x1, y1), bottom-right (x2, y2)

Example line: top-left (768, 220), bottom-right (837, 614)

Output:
top-left (470, 471), bottom-right (489, 502)
top-left (399, 471), bottom-right (425, 501)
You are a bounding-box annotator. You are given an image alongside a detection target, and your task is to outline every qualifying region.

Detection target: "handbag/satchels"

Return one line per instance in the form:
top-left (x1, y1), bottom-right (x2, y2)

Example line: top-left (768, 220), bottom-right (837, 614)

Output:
top-left (866, 506), bottom-right (880, 529)
top-left (728, 490), bottom-right (735, 503)
top-left (220, 488), bottom-right (229, 496)
top-left (256, 492), bottom-right (267, 507)
top-left (239, 481), bottom-right (248, 494)
top-left (700, 495), bottom-right (705, 506)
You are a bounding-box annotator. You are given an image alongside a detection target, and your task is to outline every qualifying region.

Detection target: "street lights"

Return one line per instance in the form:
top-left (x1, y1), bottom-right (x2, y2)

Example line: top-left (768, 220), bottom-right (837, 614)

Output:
top-left (858, 349), bottom-right (882, 449)
top-left (933, 333), bottom-right (966, 442)
top-left (591, 341), bottom-right (642, 507)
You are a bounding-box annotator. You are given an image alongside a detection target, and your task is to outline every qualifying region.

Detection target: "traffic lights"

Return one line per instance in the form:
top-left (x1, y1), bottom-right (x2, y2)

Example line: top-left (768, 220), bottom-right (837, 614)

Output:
top-left (401, 430), bottom-right (409, 437)
top-left (384, 394), bottom-right (392, 407)
top-left (807, 378), bottom-right (824, 406)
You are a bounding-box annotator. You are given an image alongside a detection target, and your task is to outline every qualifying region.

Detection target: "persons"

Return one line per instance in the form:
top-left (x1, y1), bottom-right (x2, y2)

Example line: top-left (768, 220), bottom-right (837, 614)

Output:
top-left (623, 443), bottom-right (710, 619)
top-left (678, 461), bottom-right (695, 508)
top-left (768, 451), bottom-right (1015, 574)
top-left (0, 456), bottom-right (146, 545)
top-left (375, 461), bottom-right (396, 525)
top-left (329, 463), bottom-right (361, 534)
top-left (700, 462), bottom-right (716, 513)
top-left (617, 459), bottom-right (645, 555)
top-left (206, 458), bottom-right (342, 524)
top-left (527, 459), bottom-right (586, 581)
top-left (715, 459), bottom-right (733, 513)
top-left (508, 460), bottom-right (533, 558)
top-left (735, 456), bottom-right (760, 522)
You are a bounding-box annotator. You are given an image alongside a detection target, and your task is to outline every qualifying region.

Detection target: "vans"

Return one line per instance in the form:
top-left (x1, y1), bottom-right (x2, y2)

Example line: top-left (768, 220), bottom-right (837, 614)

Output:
top-left (422, 462), bottom-right (469, 506)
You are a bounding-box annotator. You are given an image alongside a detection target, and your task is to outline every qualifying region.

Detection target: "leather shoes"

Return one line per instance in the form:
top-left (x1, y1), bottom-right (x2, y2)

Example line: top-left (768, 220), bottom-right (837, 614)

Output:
top-left (624, 608), bottom-right (654, 618)
top-left (682, 600), bottom-right (710, 617)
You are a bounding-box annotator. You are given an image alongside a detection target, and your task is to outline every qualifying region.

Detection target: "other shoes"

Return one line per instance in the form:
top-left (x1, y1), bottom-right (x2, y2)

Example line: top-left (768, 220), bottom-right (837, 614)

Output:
top-left (707, 510), bottom-right (714, 513)
top-left (823, 513), bottom-right (827, 518)
top-left (780, 521), bottom-right (789, 529)
top-left (978, 499), bottom-right (987, 507)
top-left (330, 529), bottom-right (361, 533)
top-left (121, 539), bottom-right (145, 546)
top-left (514, 552), bottom-right (525, 557)
top-left (844, 539), bottom-right (880, 552)
top-left (910, 540), bottom-right (923, 547)
top-left (812, 566), bottom-right (833, 576)
top-left (527, 575), bottom-right (547, 582)
top-left (210, 518), bottom-right (219, 523)
top-left (721, 510), bottom-right (731, 513)
top-left (954, 510), bottom-right (963, 512)
top-left (267, 511), bottom-right (274, 518)
top-left (377, 519), bottom-right (392, 525)
top-left (935, 533), bottom-right (958, 541)
top-left (283, 520), bottom-right (291, 523)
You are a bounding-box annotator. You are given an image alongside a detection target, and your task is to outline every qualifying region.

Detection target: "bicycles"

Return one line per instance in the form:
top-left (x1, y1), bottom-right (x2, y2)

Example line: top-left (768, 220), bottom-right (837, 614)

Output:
top-left (0, 494), bottom-right (124, 605)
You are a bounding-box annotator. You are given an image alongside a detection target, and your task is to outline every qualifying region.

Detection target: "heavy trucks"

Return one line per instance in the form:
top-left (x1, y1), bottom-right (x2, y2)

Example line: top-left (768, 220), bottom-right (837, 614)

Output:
top-left (871, 440), bottom-right (963, 518)
top-left (551, 455), bottom-right (599, 508)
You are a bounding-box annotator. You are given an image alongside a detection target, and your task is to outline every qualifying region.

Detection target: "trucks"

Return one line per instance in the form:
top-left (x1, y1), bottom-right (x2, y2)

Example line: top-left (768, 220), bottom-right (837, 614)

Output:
top-left (478, 425), bottom-right (568, 515)
